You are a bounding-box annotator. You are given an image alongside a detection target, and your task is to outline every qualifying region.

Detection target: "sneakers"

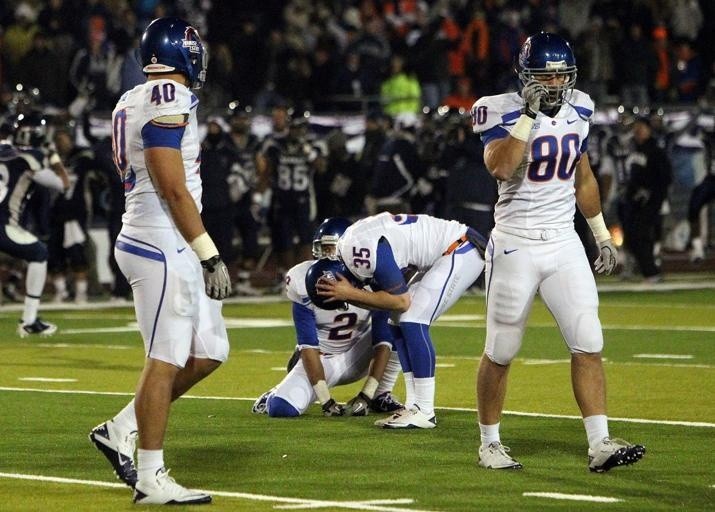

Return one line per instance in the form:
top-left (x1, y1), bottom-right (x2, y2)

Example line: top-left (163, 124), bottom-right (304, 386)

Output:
top-left (374, 409), bottom-right (411, 428)
top-left (88, 419), bottom-right (139, 492)
top-left (252, 385), bottom-right (280, 415)
top-left (370, 391), bottom-right (405, 414)
top-left (478, 441), bottom-right (524, 471)
top-left (588, 436), bottom-right (646, 474)
top-left (132, 464), bottom-right (213, 506)
top-left (17, 317), bottom-right (58, 340)
top-left (382, 404), bottom-right (437, 430)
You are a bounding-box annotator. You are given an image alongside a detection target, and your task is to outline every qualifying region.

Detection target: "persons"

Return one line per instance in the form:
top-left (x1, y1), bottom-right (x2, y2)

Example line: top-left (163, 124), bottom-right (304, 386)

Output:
top-left (88, 15), bottom-right (145, 301)
top-left (229, 217), bottom-right (251, 296)
top-left (229, 2), bottom-right (469, 218)
top-left (568, 32), bottom-right (645, 283)
top-left (0, 0), bottom-right (87, 338)
top-left (251, 217), bottom-right (405, 418)
top-left (470, 0), bottom-right (647, 31)
top-left (305, 211), bottom-right (492, 429)
top-left (251, 216), bottom-right (304, 298)
top-left (646, 0), bottom-right (715, 280)
top-left (189, 14), bottom-right (228, 255)
top-left (471, 135), bottom-right (497, 234)
top-left (87, 0), bottom-right (228, 15)
top-left (470, 32), bottom-right (522, 97)
top-left (87, 15), bottom-right (229, 506)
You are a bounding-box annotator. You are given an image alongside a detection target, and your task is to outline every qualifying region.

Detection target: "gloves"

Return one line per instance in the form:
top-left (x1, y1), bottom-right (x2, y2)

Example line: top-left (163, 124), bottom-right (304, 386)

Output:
top-left (199, 254), bottom-right (234, 301)
top-left (521, 79), bottom-right (549, 120)
top-left (321, 398), bottom-right (343, 418)
top-left (342, 391), bottom-right (371, 418)
top-left (594, 239), bottom-right (620, 276)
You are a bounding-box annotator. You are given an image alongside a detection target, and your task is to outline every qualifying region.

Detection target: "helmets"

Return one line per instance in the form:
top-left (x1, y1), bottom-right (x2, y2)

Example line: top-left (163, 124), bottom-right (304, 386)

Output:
top-left (11, 108), bottom-right (49, 148)
top-left (312, 216), bottom-right (356, 260)
top-left (140, 14), bottom-right (211, 90)
top-left (286, 104), bottom-right (311, 137)
top-left (305, 255), bottom-right (357, 312)
top-left (512, 33), bottom-right (579, 109)
top-left (223, 98), bottom-right (254, 132)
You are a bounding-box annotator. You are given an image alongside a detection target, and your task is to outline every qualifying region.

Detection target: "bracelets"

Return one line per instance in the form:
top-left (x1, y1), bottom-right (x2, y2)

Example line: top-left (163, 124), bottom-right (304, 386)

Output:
top-left (470, 32), bottom-right (647, 474)
